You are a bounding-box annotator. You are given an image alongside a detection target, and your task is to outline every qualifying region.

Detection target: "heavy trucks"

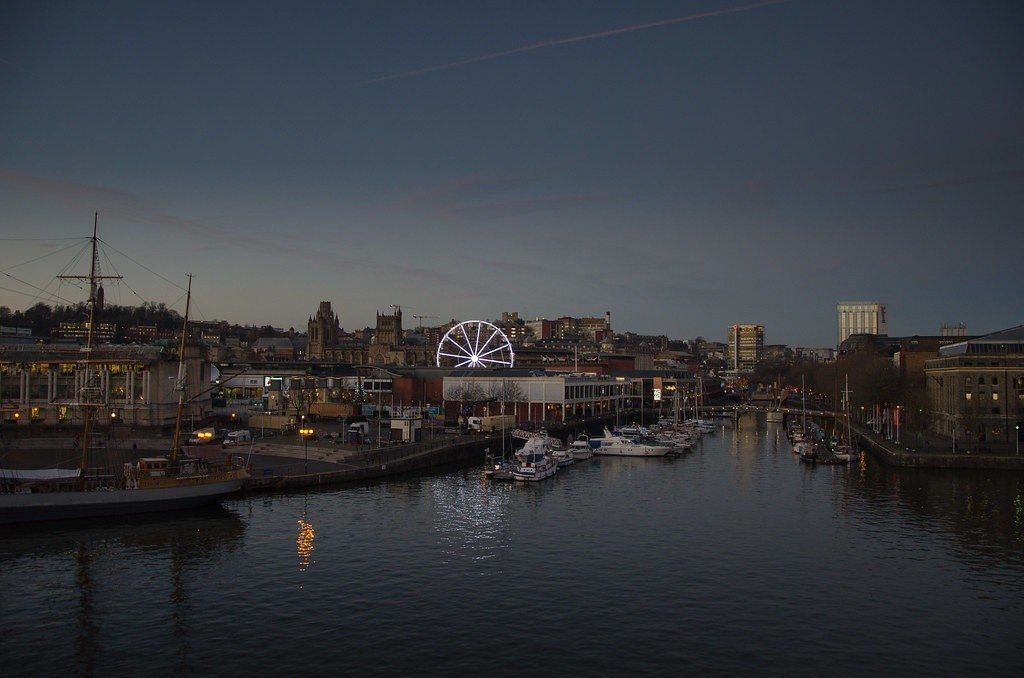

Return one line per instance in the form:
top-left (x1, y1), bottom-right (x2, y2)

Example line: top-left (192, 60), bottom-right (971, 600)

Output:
top-left (431, 416), bottom-right (482, 435)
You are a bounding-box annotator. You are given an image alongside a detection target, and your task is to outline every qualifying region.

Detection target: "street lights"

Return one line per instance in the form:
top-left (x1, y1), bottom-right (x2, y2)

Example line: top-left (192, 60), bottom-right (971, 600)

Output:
top-left (299, 429), bottom-right (314, 475)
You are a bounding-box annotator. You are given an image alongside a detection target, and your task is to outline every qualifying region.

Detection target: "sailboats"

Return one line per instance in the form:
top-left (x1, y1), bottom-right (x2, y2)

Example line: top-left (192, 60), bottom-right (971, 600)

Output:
top-left (482, 377), bottom-right (513, 480)
top-left (792, 375), bottom-right (812, 439)
top-left (658, 377), bottom-right (717, 434)
top-left (827, 373), bottom-right (861, 462)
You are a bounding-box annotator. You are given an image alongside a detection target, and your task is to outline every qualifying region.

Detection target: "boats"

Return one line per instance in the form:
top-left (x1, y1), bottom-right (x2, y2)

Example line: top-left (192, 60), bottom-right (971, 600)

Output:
top-left (551, 450), bottom-right (575, 467)
top-left (0, 211), bottom-right (249, 521)
top-left (589, 425), bottom-right (697, 456)
top-left (793, 441), bottom-right (823, 462)
top-left (569, 431), bottom-right (593, 460)
top-left (513, 406), bottom-right (559, 482)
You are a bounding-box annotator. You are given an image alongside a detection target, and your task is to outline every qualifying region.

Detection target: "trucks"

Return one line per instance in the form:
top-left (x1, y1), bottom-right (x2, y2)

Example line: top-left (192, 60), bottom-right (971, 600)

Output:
top-left (348, 422), bottom-right (369, 436)
top-left (189, 427), bottom-right (216, 444)
top-left (222, 430), bottom-right (249, 445)
